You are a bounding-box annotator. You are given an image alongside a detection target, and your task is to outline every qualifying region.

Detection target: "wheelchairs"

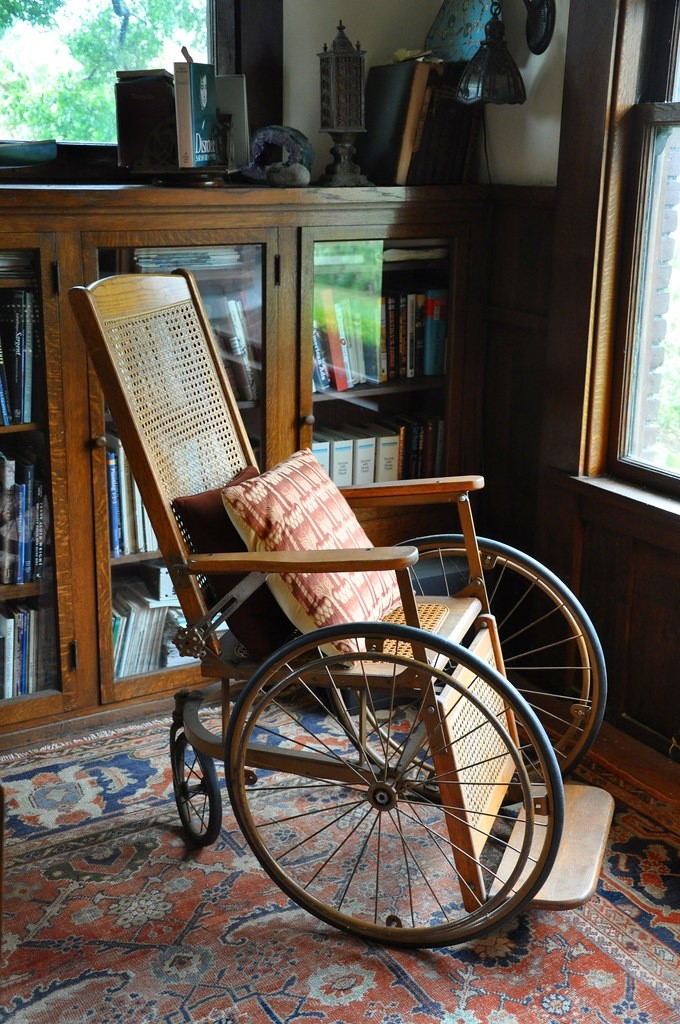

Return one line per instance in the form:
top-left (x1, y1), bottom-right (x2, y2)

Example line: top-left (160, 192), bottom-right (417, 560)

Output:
top-left (68, 267), bottom-right (610, 947)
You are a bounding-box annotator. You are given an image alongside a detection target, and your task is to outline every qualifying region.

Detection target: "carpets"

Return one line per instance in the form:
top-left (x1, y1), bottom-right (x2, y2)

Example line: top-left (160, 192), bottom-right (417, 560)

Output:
top-left (0, 684), bottom-right (680, 1024)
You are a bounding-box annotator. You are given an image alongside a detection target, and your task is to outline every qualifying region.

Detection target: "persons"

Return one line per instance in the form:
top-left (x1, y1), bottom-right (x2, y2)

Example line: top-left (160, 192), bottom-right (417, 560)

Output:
top-left (194, 73), bottom-right (216, 140)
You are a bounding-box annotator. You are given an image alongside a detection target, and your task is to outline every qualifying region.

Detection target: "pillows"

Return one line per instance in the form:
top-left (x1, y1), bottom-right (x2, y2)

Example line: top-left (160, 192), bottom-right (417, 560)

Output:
top-left (223, 446), bottom-right (415, 666)
top-left (174, 466), bottom-right (298, 666)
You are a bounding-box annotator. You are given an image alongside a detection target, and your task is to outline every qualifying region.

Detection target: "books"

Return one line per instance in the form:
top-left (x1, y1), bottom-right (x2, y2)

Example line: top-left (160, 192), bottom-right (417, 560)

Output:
top-left (205, 289), bottom-right (265, 403)
top-left (111, 577), bottom-right (229, 679)
top-left (311, 239), bottom-right (447, 392)
top-left (173, 61), bottom-right (221, 169)
top-left (114, 68), bottom-right (177, 168)
top-left (105, 426), bottom-right (159, 557)
top-left (0, 278), bottom-right (45, 426)
top-left (351, 59), bottom-right (483, 186)
top-left (0, 445), bottom-right (50, 587)
top-left (311, 406), bottom-right (447, 487)
top-left (0, 601), bottom-right (56, 700)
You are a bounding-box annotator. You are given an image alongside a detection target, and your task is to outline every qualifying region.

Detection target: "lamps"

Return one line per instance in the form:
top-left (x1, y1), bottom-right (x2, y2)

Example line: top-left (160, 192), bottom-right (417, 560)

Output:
top-left (314, 20), bottom-right (376, 186)
top-left (455, 0), bottom-right (556, 105)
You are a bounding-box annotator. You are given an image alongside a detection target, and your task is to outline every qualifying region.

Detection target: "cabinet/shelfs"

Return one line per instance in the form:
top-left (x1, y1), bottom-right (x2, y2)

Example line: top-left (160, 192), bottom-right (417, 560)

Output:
top-left (0, 184), bottom-right (558, 752)
